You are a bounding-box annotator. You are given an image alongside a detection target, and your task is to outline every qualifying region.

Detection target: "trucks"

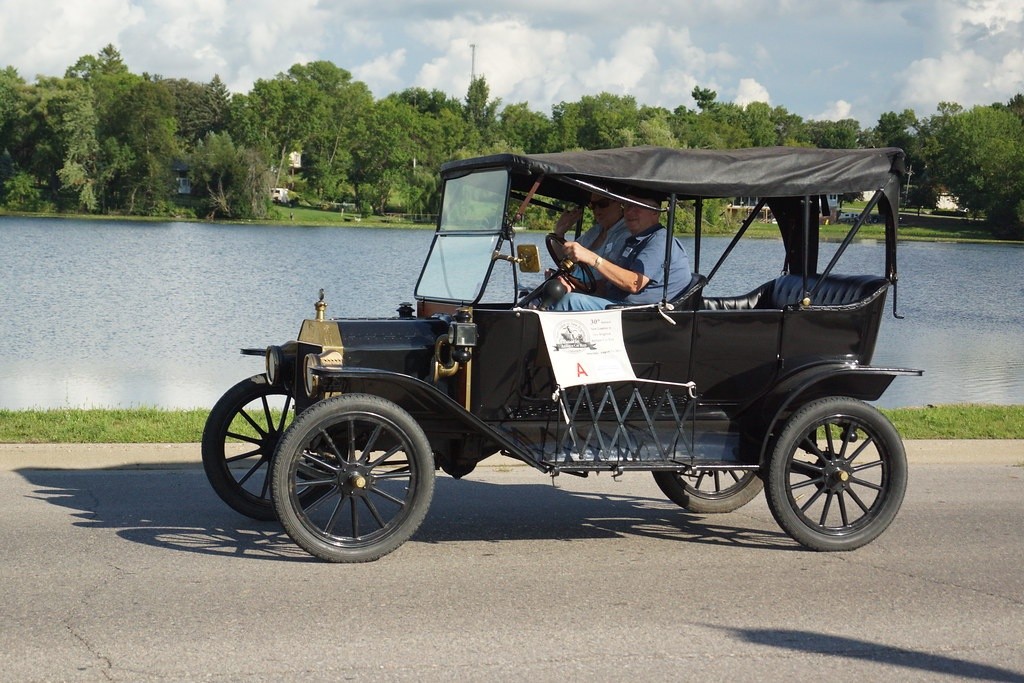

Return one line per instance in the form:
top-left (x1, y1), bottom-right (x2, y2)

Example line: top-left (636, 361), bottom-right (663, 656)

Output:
top-left (838, 213), bottom-right (860, 223)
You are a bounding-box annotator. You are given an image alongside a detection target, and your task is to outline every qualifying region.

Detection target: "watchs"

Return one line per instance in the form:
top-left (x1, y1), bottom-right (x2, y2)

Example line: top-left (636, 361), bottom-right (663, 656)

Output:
top-left (592, 257), bottom-right (602, 271)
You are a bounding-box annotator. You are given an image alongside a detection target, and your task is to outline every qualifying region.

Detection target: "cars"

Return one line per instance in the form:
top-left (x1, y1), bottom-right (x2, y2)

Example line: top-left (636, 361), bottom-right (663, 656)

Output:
top-left (871, 214), bottom-right (903, 224)
top-left (201, 146), bottom-right (926, 567)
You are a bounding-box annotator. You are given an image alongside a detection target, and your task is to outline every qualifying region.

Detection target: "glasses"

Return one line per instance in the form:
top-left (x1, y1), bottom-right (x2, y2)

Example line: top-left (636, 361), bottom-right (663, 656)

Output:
top-left (587, 198), bottom-right (609, 210)
top-left (620, 203), bottom-right (638, 209)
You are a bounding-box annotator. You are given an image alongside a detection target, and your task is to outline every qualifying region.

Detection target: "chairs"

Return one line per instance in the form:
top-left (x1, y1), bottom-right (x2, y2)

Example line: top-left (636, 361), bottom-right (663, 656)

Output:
top-left (606, 272), bottom-right (709, 312)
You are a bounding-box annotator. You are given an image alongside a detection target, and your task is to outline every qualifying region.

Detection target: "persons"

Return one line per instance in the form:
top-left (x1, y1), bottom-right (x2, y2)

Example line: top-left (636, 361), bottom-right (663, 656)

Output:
top-left (523, 187), bottom-right (692, 313)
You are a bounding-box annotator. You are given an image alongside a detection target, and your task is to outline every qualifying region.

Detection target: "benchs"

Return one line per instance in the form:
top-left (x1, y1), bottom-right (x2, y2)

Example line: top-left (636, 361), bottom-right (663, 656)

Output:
top-left (699, 273), bottom-right (890, 311)
